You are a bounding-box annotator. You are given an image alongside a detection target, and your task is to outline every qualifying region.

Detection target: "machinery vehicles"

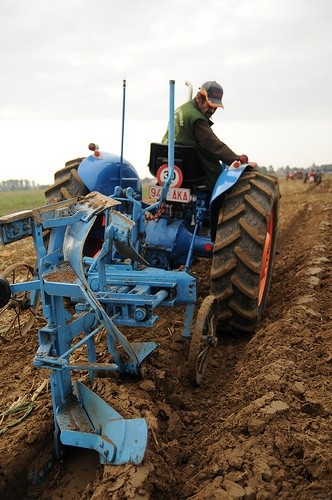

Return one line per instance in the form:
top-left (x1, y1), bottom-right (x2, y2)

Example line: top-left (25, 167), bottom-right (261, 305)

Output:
top-left (0, 79), bottom-right (280, 498)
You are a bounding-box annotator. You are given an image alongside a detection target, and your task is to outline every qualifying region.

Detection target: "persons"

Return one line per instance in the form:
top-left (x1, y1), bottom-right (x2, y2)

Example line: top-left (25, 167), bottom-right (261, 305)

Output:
top-left (303, 168), bottom-right (326, 185)
top-left (161, 80), bottom-right (258, 210)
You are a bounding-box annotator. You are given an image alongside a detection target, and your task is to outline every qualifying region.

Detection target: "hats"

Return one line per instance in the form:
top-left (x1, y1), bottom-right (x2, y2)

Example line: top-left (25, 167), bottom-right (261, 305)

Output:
top-left (199, 81), bottom-right (224, 109)
